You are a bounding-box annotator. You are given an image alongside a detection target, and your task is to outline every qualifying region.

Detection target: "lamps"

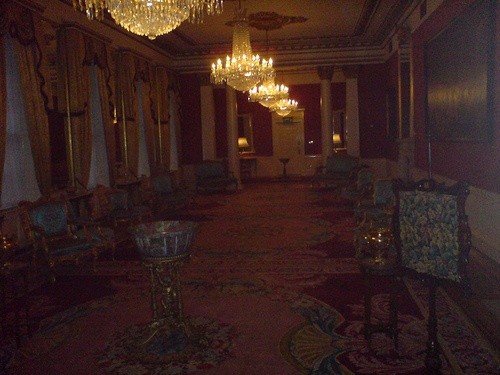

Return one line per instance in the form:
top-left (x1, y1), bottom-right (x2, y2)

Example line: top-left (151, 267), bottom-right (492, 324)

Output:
top-left (334, 135), bottom-right (342, 148)
top-left (247, 24), bottom-right (289, 108)
top-left (211, 0), bottom-right (276, 93)
top-left (74, 0), bottom-right (223, 40)
top-left (268, 71), bottom-right (298, 116)
top-left (238, 138), bottom-right (249, 153)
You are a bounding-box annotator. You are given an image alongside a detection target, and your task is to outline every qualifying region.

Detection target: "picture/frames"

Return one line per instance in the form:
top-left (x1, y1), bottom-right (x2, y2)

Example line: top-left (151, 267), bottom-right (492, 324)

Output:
top-left (423, 0), bottom-right (494, 144)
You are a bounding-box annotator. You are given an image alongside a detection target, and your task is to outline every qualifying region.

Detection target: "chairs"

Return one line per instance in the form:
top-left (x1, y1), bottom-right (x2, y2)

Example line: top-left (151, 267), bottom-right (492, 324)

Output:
top-left (0, 166), bottom-right (194, 302)
top-left (196, 160), bottom-right (238, 194)
